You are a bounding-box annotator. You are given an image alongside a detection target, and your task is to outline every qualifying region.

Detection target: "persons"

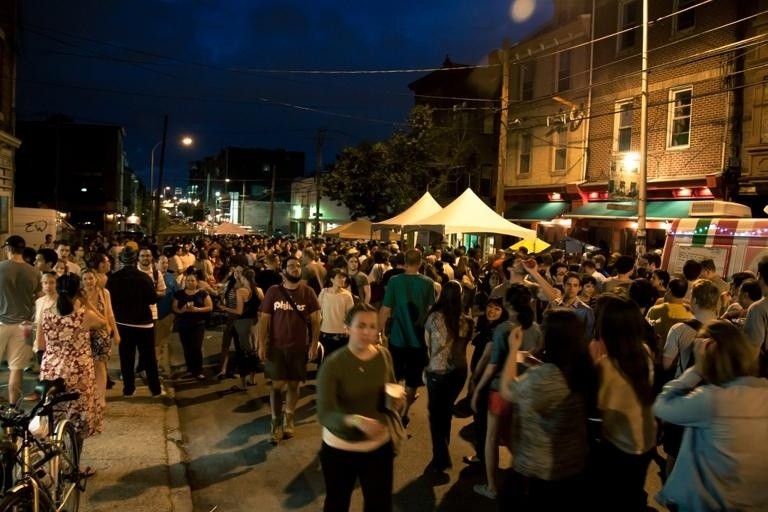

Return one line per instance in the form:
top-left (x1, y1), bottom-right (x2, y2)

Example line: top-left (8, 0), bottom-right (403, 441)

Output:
top-left (318, 235), bottom-right (768, 511)
top-left (1, 220), bottom-right (323, 479)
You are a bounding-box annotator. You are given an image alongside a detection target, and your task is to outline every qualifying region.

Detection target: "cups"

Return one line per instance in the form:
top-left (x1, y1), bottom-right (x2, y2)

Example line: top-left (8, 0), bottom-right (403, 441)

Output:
top-left (523, 260), bottom-right (536, 269)
top-left (187, 302), bottom-right (194, 312)
top-left (385, 382), bottom-right (404, 412)
top-left (516, 350), bottom-right (529, 363)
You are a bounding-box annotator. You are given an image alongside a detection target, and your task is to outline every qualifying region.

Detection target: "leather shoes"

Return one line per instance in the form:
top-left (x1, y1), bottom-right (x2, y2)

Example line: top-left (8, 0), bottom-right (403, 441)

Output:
top-left (23, 393), bottom-right (40, 400)
top-left (464, 456), bottom-right (483, 465)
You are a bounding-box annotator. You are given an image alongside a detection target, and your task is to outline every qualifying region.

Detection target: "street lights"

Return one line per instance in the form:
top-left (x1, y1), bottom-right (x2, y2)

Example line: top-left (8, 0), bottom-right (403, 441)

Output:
top-left (147, 136), bottom-right (195, 238)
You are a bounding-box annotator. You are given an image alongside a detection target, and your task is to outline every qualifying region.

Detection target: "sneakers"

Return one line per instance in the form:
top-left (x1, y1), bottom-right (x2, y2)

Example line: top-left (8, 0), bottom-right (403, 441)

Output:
top-left (424, 459), bottom-right (452, 475)
top-left (283, 412), bottom-right (295, 438)
top-left (268, 422), bottom-right (281, 443)
top-left (107, 369), bottom-right (257, 399)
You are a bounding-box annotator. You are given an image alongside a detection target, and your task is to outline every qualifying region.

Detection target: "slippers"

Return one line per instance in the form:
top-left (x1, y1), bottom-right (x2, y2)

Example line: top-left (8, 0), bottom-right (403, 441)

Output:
top-left (473, 483), bottom-right (497, 499)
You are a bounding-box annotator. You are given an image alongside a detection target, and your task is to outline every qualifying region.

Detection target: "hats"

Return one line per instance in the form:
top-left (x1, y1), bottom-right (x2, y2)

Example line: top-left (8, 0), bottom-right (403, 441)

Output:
top-left (120, 246), bottom-right (138, 264)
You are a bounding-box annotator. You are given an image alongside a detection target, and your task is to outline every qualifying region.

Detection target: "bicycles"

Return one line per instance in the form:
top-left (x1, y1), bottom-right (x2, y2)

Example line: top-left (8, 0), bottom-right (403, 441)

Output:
top-left (0, 380), bottom-right (86, 512)
